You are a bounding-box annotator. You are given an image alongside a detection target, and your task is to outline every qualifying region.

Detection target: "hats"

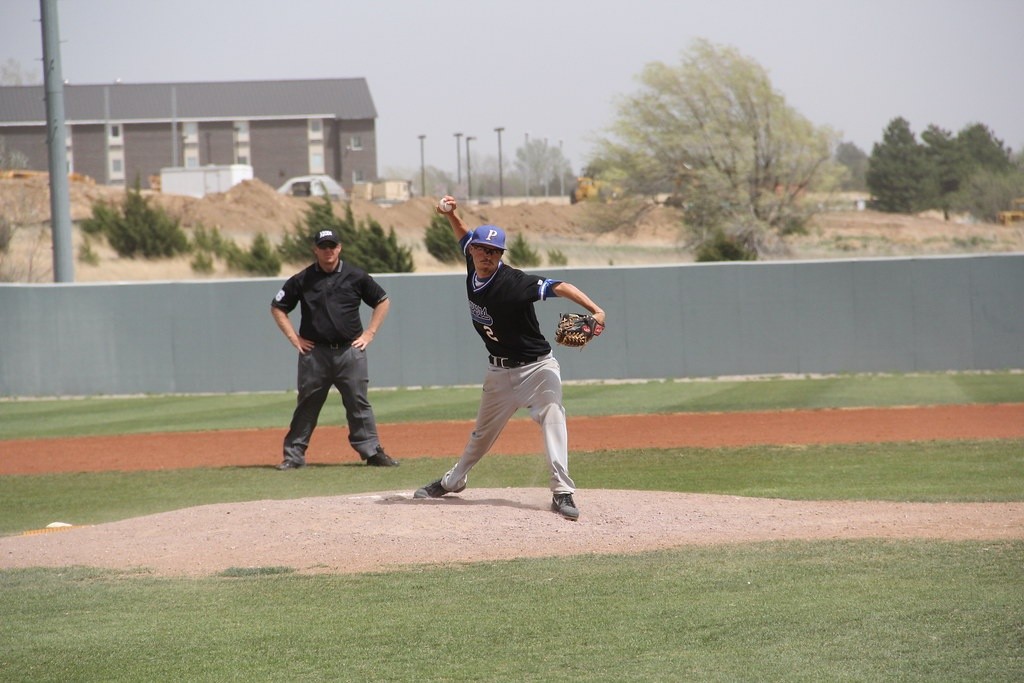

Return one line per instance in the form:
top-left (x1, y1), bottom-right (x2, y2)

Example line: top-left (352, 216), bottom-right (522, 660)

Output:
top-left (314, 229), bottom-right (339, 246)
top-left (470, 225), bottom-right (507, 251)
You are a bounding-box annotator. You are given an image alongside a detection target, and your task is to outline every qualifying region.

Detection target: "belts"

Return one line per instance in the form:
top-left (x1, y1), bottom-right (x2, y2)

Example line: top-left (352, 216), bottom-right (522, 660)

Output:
top-left (488, 355), bottom-right (537, 369)
top-left (317, 339), bottom-right (353, 350)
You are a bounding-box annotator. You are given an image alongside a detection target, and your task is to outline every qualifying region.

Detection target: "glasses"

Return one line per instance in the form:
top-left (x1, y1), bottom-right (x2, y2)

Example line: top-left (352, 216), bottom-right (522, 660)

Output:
top-left (472, 244), bottom-right (501, 255)
top-left (317, 242), bottom-right (337, 250)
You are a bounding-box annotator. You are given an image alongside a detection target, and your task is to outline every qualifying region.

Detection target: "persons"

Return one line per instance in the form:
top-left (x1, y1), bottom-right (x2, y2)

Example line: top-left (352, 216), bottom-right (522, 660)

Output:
top-left (269, 228), bottom-right (401, 470)
top-left (413, 194), bottom-right (607, 522)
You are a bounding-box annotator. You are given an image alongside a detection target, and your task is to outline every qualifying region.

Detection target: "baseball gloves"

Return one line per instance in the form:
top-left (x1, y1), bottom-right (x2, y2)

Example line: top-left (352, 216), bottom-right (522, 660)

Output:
top-left (555, 312), bottom-right (605, 347)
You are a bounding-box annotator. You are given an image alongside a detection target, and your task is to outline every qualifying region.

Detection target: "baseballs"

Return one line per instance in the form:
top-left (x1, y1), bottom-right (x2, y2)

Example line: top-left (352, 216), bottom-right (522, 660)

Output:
top-left (439, 198), bottom-right (452, 212)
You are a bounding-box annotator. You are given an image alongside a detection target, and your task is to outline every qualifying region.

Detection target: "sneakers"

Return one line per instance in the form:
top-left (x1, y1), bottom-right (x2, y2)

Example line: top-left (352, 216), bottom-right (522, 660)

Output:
top-left (414, 478), bottom-right (466, 498)
top-left (367, 446), bottom-right (400, 467)
top-left (550, 493), bottom-right (579, 520)
top-left (276, 460), bottom-right (301, 470)
